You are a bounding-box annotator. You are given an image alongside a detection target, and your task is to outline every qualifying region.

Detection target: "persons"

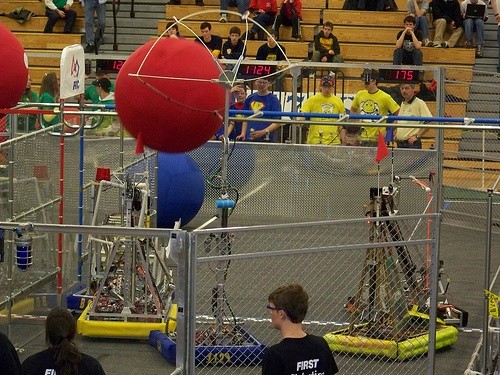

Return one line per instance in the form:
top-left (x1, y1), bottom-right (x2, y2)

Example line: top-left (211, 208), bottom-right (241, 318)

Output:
top-left (75, 68), bottom-right (115, 106)
top-left (7, 74), bottom-right (40, 132)
top-left (261, 283), bottom-right (339, 375)
top-left (236, 73), bottom-right (281, 142)
top-left (349, 68), bottom-right (401, 147)
top-left (43, 0), bottom-right (77, 33)
top-left (408, 0), bottom-right (500, 71)
top-left (91, 77), bottom-right (116, 111)
top-left (211, 84), bottom-right (248, 141)
top-left (165, 0), bottom-right (303, 60)
top-left (0, 306), bottom-right (106, 375)
top-left (307, 21), bottom-right (344, 80)
top-left (294, 76), bottom-right (348, 146)
top-left (79, 0), bottom-right (108, 54)
top-left (392, 83), bottom-right (433, 149)
top-left (35, 72), bottom-right (61, 131)
top-left (392, 15), bottom-right (425, 66)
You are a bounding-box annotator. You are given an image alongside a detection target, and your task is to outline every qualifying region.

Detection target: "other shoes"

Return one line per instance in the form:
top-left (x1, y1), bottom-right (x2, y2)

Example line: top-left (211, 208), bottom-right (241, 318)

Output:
top-left (309, 71), bottom-right (315, 78)
top-left (245, 30), bottom-right (254, 39)
top-left (329, 70), bottom-right (336, 78)
top-left (433, 40), bottom-right (449, 48)
top-left (84, 43), bottom-right (96, 53)
top-left (424, 40), bottom-right (433, 47)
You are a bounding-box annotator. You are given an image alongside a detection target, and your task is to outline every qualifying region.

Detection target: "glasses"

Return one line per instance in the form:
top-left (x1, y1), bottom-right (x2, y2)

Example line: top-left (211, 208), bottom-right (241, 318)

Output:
top-left (266, 304), bottom-right (285, 313)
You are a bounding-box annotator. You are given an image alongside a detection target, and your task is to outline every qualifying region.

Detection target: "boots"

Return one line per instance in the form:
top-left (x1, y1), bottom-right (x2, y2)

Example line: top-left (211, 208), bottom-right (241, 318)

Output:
top-left (465, 41), bottom-right (483, 57)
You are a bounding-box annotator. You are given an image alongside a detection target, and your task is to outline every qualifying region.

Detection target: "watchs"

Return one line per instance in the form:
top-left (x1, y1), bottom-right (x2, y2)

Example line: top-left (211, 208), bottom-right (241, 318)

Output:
top-left (266, 129), bottom-right (269, 136)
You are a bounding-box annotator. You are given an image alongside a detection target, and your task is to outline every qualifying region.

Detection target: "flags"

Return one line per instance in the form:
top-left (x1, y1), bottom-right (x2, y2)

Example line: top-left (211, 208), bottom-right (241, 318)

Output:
top-left (376, 132), bottom-right (390, 162)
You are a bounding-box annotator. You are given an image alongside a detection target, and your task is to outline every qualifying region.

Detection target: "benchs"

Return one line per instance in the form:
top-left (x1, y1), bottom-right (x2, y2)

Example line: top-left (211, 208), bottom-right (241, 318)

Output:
top-left (0, 0), bottom-right (482, 191)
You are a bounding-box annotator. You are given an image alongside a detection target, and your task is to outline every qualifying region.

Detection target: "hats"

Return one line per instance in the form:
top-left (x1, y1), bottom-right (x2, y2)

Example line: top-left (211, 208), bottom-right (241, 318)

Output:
top-left (92, 78), bottom-right (111, 91)
top-left (320, 75), bottom-right (334, 86)
top-left (95, 66), bottom-right (110, 74)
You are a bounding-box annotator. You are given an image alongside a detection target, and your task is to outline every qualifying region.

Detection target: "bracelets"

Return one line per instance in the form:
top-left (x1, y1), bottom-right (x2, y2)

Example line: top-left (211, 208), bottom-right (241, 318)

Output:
top-left (417, 135), bottom-right (420, 139)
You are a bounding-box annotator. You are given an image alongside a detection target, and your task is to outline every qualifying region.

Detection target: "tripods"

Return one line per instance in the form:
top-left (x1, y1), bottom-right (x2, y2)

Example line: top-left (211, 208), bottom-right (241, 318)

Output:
top-left (345, 186), bottom-right (429, 335)
